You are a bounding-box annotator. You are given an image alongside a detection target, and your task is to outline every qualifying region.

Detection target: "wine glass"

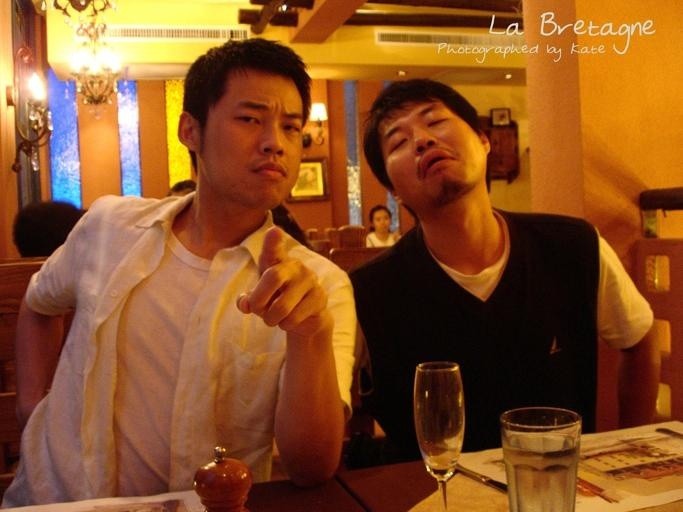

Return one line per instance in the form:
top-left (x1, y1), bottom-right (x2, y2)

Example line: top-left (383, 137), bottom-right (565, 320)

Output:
top-left (413, 361), bottom-right (465, 512)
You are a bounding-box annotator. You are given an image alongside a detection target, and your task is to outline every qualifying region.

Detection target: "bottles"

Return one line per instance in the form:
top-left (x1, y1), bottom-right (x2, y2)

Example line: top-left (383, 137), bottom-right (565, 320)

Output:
top-left (193, 447), bottom-right (250, 512)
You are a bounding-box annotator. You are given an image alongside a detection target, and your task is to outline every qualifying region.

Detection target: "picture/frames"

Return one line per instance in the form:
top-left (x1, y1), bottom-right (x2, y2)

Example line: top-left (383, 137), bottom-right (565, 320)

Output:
top-left (284, 155), bottom-right (332, 203)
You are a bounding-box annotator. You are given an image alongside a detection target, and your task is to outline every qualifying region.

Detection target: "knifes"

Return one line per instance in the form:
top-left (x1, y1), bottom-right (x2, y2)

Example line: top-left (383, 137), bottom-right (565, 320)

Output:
top-left (454, 464), bottom-right (507, 494)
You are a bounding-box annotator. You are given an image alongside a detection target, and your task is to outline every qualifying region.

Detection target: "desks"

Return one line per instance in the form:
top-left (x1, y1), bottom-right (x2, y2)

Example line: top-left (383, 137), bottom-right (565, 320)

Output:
top-left (337, 418), bottom-right (683, 512)
top-left (245, 475), bottom-right (368, 512)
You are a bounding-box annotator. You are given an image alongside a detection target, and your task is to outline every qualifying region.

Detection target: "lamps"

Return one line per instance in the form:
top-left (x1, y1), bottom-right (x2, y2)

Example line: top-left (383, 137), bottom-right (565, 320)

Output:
top-left (7, 74), bottom-right (54, 173)
top-left (54, 0), bottom-right (127, 104)
top-left (302, 102), bottom-right (329, 148)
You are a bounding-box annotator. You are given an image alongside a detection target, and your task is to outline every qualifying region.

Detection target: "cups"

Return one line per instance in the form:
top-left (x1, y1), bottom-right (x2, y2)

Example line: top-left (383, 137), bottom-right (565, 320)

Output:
top-left (499, 406), bottom-right (582, 512)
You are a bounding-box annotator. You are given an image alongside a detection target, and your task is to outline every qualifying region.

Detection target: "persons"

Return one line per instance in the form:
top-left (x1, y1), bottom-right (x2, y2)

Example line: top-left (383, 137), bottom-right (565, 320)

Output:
top-left (167, 180), bottom-right (198, 201)
top-left (345, 77), bottom-right (663, 465)
top-left (0, 41), bottom-right (356, 509)
top-left (366, 205), bottom-right (398, 248)
top-left (271, 203), bottom-right (315, 253)
top-left (12, 200), bottom-right (80, 257)
